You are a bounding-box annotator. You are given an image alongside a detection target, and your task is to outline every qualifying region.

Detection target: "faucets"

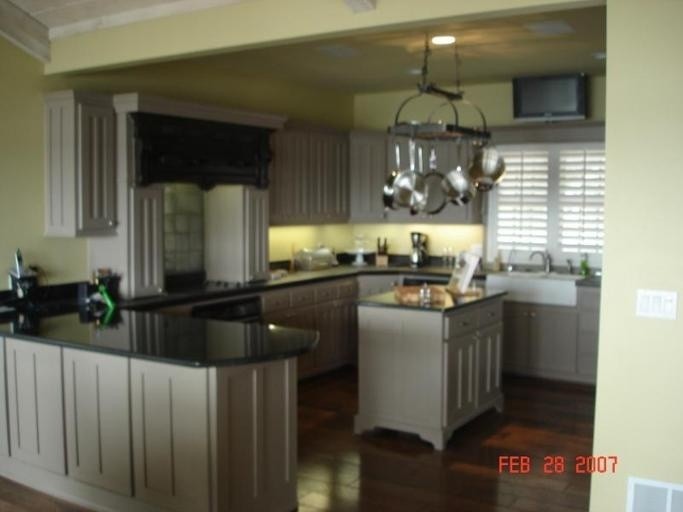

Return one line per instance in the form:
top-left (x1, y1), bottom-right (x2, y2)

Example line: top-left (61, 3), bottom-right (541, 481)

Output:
top-left (529, 249), bottom-right (552, 274)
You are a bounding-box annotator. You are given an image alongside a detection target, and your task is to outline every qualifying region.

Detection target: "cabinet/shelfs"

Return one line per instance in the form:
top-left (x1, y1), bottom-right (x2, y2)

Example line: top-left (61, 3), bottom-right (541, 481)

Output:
top-left (262, 280), bottom-right (361, 381)
top-left (89, 115), bottom-right (166, 300)
top-left (504, 301), bottom-right (576, 382)
top-left (204, 186), bottom-right (270, 282)
top-left (45, 103), bottom-right (119, 239)
top-left (0, 336), bottom-right (299, 511)
top-left (270, 137), bottom-right (349, 226)
top-left (351, 135), bottom-right (485, 225)
top-left (354, 297), bottom-right (506, 449)
top-left (577, 287), bottom-right (600, 383)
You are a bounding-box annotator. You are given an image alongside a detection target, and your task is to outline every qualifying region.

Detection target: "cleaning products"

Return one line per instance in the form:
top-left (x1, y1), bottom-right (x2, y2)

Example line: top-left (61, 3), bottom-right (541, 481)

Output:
top-left (580, 253), bottom-right (588, 275)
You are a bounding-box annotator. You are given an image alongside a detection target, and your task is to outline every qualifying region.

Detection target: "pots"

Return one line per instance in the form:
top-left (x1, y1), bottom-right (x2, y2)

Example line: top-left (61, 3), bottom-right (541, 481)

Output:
top-left (470, 135), bottom-right (508, 194)
top-left (393, 119), bottom-right (429, 211)
top-left (441, 136), bottom-right (476, 208)
top-left (378, 131), bottom-right (402, 212)
top-left (423, 134), bottom-right (447, 217)
